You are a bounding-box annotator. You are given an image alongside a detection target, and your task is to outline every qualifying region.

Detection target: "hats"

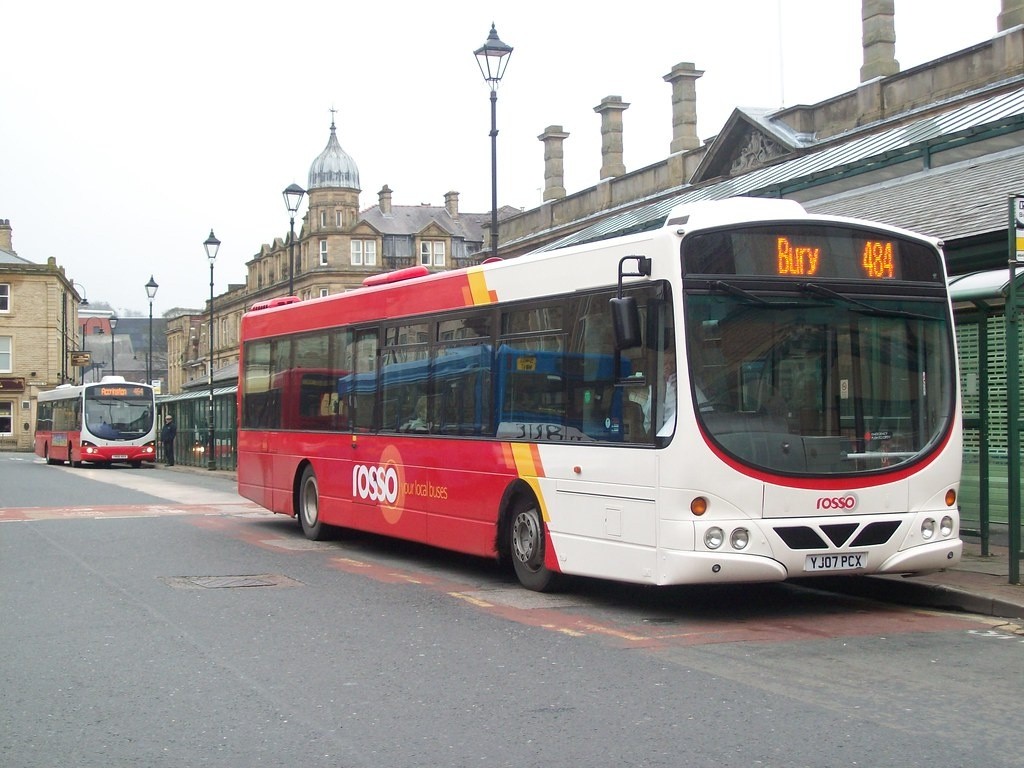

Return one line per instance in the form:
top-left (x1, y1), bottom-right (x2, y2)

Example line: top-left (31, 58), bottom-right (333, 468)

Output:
top-left (165, 415), bottom-right (172, 420)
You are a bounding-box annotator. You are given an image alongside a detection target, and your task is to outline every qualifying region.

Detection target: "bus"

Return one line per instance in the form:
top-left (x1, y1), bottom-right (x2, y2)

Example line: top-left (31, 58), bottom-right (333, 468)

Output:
top-left (237, 198), bottom-right (964, 593)
top-left (331, 340), bottom-right (633, 444)
top-left (243, 366), bottom-right (350, 431)
top-left (33, 375), bottom-right (162, 469)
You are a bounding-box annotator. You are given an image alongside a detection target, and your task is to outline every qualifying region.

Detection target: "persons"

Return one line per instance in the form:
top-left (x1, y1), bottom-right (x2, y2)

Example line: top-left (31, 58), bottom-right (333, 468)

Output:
top-left (444, 387), bottom-right (459, 423)
top-left (507, 367), bottom-right (648, 440)
top-left (162, 415), bottom-right (177, 466)
top-left (626, 341), bottom-right (714, 440)
top-left (406, 394), bottom-right (440, 432)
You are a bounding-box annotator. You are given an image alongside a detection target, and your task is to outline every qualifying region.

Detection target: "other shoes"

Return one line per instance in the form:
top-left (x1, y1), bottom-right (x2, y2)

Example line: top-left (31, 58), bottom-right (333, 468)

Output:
top-left (164, 463), bottom-right (173, 467)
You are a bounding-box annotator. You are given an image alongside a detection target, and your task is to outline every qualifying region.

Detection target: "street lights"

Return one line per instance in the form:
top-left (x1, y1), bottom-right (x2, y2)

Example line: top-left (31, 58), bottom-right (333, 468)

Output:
top-left (473, 20), bottom-right (514, 263)
top-left (144, 274), bottom-right (159, 386)
top-left (203, 228), bottom-right (221, 472)
top-left (81, 315), bottom-right (105, 387)
top-left (108, 313), bottom-right (118, 377)
top-left (61, 282), bottom-right (89, 384)
top-left (281, 181), bottom-right (307, 298)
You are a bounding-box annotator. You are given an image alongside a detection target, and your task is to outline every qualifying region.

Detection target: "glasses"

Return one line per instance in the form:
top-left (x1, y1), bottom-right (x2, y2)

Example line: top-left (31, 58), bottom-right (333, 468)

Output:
top-left (166, 418), bottom-right (172, 420)
